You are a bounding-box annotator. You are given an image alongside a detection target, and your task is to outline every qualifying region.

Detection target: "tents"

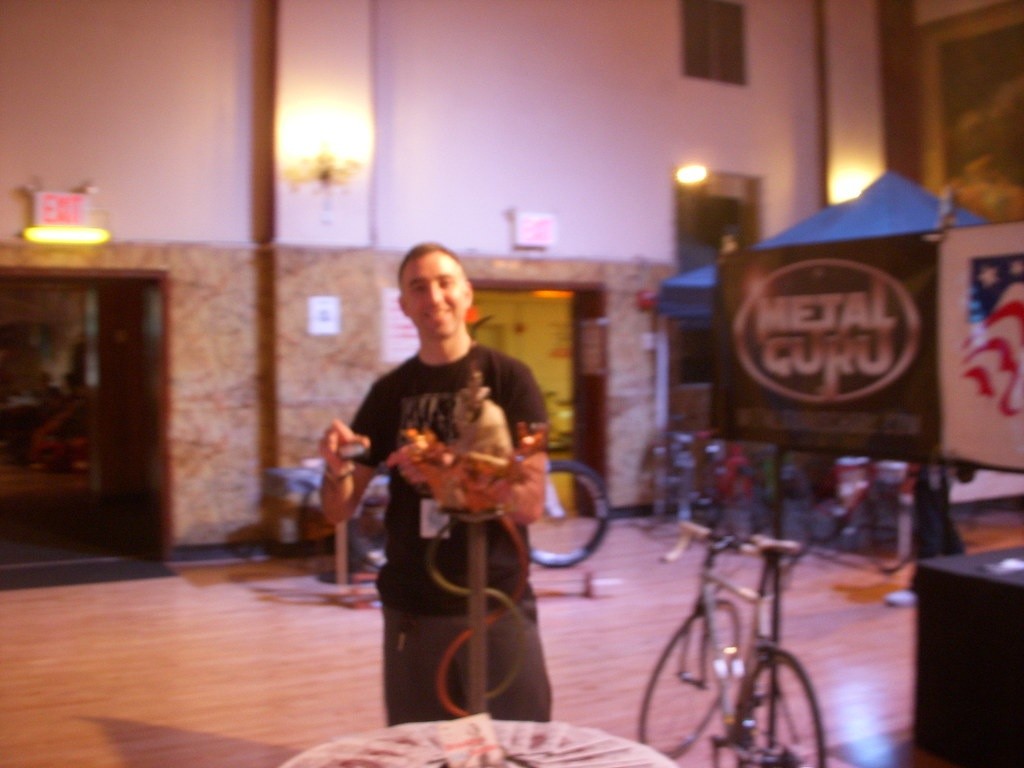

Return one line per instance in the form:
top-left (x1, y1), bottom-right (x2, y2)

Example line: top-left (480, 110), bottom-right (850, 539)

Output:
top-left (654, 167), bottom-right (977, 563)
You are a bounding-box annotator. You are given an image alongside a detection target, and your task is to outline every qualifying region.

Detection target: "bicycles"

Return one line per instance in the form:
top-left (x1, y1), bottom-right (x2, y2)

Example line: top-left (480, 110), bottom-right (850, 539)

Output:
top-left (638, 522), bottom-right (825, 768)
top-left (354, 440), bottom-right (610, 568)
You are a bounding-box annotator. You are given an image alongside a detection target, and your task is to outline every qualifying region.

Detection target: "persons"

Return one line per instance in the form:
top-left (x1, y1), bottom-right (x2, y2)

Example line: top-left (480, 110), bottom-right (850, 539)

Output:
top-left (318, 242), bottom-right (548, 721)
top-left (30, 371), bottom-right (90, 469)
top-left (883, 468), bottom-right (965, 610)
